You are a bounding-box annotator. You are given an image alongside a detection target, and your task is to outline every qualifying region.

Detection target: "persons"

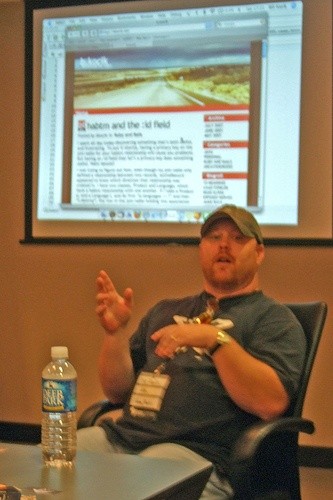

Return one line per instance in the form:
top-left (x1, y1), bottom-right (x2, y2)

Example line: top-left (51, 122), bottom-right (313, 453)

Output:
top-left (37, 204), bottom-right (305, 500)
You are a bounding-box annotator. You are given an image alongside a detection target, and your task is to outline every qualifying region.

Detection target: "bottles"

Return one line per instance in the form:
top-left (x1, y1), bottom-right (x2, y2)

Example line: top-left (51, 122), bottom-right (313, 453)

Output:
top-left (41, 345), bottom-right (78, 465)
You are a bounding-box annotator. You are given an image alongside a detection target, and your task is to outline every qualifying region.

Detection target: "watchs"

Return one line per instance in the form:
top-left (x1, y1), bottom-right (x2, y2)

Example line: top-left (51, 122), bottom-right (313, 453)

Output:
top-left (209, 330), bottom-right (231, 355)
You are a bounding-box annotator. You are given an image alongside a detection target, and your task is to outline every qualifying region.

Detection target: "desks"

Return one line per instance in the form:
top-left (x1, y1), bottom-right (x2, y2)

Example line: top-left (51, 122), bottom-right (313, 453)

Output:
top-left (0, 442), bottom-right (215, 500)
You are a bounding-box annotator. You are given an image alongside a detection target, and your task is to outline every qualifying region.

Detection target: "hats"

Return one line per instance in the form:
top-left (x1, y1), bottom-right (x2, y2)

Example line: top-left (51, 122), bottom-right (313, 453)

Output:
top-left (200, 203), bottom-right (264, 245)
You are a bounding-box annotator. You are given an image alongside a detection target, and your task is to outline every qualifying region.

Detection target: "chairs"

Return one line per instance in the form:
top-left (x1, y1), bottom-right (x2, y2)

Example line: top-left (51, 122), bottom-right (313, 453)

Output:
top-left (78, 301), bottom-right (327, 500)
